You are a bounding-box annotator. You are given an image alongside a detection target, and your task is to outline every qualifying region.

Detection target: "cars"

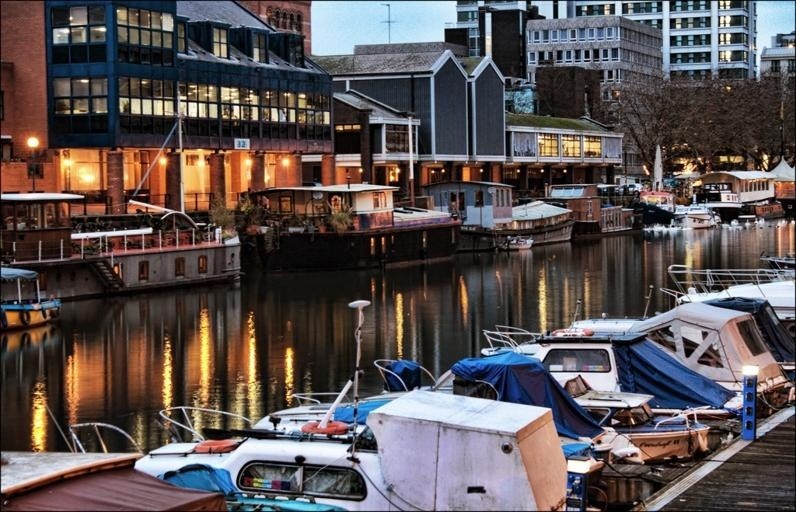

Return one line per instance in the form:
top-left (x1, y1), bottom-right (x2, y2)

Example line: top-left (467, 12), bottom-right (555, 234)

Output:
top-left (597, 181), bottom-right (732, 204)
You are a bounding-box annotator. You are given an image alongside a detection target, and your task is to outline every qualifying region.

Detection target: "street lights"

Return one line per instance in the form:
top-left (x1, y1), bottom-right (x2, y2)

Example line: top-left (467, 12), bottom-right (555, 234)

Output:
top-left (26, 136), bottom-right (40, 194)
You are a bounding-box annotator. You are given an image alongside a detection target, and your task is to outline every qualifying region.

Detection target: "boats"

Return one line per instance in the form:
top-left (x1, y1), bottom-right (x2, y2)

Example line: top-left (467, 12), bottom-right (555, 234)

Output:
top-left (500, 237), bottom-right (535, 249)
top-left (673, 207), bottom-right (720, 229)
